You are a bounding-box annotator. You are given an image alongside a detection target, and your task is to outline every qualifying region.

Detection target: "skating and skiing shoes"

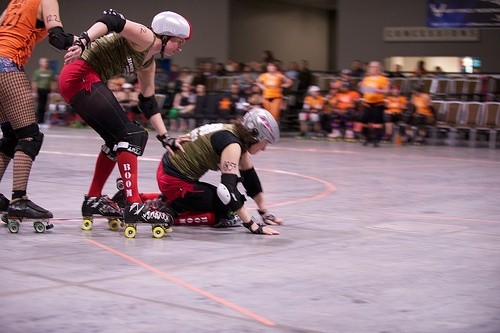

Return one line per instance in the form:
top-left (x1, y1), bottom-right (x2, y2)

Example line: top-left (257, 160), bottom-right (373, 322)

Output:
top-left (79, 176), bottom-right (178, 238)
top-left (1, 194), bottom-right (54, 233)
top-left (294, 131), bottom-right (426, 146)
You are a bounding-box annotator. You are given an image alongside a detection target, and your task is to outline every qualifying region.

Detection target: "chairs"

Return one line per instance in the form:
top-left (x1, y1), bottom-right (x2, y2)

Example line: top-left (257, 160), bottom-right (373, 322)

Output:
top-left (195, 73), bottom-right (500, 148)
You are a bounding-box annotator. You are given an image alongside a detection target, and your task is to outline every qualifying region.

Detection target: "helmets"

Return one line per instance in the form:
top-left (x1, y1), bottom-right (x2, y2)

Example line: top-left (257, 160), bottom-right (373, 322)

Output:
top-left (342, 80), bottom-right (352, 88)
top-left (390, 83), bottom-right (400, 90)
top-left (413, 84), bottom-right (425, 92)
top-left (238, 108), bottom-right (280, 146)
top-left (151, 11), bottom-right (192, 41)
top-left (308, 86), bottom-right (320, 93)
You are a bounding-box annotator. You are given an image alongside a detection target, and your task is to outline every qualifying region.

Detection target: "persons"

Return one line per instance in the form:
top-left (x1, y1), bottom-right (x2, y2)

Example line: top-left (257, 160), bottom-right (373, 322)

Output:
top-left (0, 0), bottom-right (81, 234)
top-left (112, 107), bottom-right (284, 236)
top-left (256, 61), bottom-right (294, 122)
top-left (58, 9), bottom-right (192, 238)
top-left (30, 49), bottom-right (445, 149)
top-left (357, 59), bottom-right (391, 148)
top-left (32, 57), bottom-right (55, 124)
